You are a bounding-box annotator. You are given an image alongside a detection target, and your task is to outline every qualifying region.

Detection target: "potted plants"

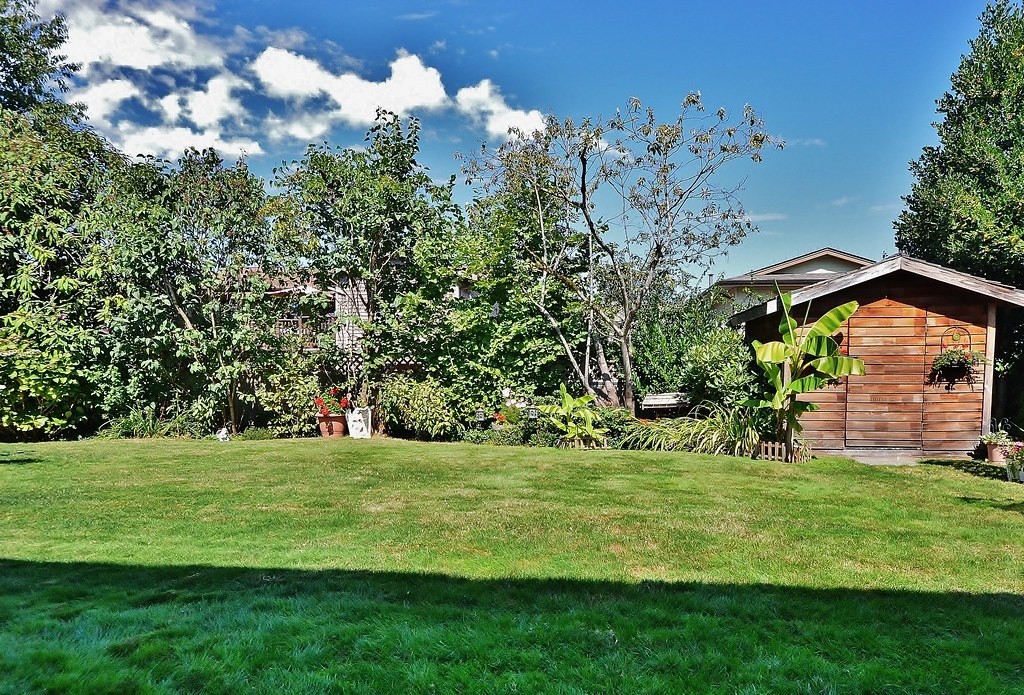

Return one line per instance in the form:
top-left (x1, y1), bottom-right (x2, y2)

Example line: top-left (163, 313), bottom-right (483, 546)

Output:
top-left (980, 432), bottom-right (1014, 462)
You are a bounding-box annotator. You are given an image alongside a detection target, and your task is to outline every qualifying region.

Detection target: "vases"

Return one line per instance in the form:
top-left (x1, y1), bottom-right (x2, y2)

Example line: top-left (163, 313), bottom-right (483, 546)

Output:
top-left (941, 365), bottom-right (971, 379)
top-left (491, 424), bottom-right (519, 445)
top-left (1005, 457), bottom-right (1023, 483)
top-left (315, 412), bottom-right (347, 437)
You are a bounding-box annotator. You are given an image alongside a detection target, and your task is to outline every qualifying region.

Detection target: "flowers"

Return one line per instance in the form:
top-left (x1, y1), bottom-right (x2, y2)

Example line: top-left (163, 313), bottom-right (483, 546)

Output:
top-left (315, 388), bottom-right (347, 416)
top-left (926, 343), bottom-right (993, 390)
top-left (1000, 442), bottom-right (1023, 479)
top-left (491, 388), bottom-right (525, 426)
